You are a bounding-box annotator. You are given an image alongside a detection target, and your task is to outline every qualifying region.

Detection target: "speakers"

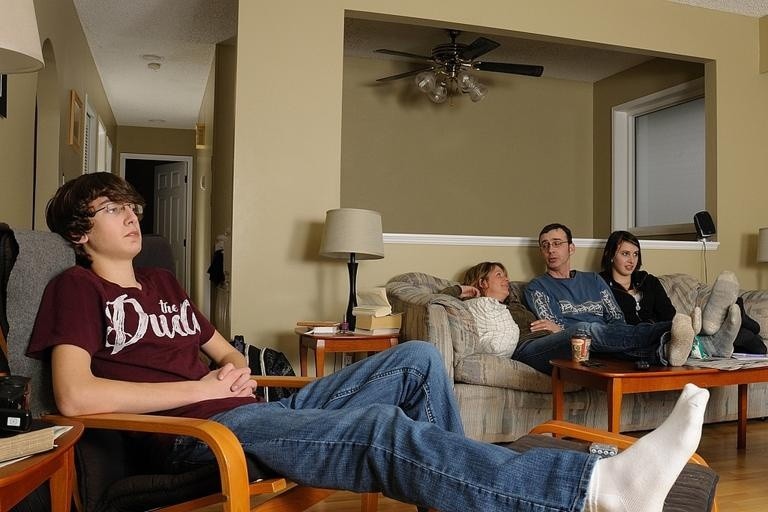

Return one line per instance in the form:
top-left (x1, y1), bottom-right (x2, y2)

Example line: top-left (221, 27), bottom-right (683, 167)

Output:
top-left (694, 211), bottom-right (716, 241)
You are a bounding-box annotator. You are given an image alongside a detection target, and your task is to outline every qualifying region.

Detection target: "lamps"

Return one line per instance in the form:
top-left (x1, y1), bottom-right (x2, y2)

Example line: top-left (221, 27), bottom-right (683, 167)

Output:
top-left (195, 121), bottom-right (207, 150)
top-left (0, 0), bottom-right (43, 379)
top-left (757, 227), bottom-right (767, 263)
top-left (415, 64), bottom-right (486, 108)
top-left (318, 206), bottom-right (385, 331)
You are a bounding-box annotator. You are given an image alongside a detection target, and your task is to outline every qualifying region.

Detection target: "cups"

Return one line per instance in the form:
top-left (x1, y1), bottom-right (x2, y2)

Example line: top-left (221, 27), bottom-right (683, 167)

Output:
top-left (569, 334), bottom-right (591, 363)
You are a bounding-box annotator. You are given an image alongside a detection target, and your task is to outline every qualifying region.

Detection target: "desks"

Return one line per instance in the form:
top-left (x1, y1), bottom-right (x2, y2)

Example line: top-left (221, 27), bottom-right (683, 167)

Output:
top-left (294, 325), bottom-right (400, 383)
top-left (0, 416), bottom-right (86, 512)
top-left (546, 348), bottom-right (766, 457)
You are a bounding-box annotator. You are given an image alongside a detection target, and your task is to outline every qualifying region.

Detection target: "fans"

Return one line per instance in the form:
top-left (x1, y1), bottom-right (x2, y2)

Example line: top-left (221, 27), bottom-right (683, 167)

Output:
top-left (371, 36), bottom-right (544, 84)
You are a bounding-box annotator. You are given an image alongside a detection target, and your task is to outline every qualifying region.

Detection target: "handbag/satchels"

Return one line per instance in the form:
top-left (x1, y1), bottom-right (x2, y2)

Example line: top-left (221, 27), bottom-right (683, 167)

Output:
top-left (209, 336), bottom-right (296, 402)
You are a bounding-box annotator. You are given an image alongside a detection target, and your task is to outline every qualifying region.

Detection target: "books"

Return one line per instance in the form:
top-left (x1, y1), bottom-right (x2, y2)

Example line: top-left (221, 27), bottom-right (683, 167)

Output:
top-left (296, 320), bottom-right (340, 327)
top-left (354, 313), bottom-right (402, 330)
top-left (313, 326), bottom-right (339, 334)
top-left (351, 287), bottom-right (392, 317)
top-left (353, 328), bottom-right (400, 336)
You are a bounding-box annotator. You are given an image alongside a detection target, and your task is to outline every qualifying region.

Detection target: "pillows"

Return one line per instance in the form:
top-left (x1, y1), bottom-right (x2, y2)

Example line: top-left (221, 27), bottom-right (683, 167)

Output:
top-left (460, 296), bottom-right (520, 359)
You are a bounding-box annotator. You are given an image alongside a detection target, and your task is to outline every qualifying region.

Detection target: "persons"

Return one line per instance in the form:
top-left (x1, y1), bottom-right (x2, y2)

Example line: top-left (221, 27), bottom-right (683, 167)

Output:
top-left (24, 172), bottom-right (712, 511)
top-left (597, 229), bottom-right (741, 360)
top-left (438, 261), bottom-right (703, 378)
top-left (520, 222), bottom-right (695, 369)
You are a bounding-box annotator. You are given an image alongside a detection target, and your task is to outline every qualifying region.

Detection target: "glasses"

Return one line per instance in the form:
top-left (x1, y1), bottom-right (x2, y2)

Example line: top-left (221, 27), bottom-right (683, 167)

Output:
top-left (96, 203), bottom-right (143, 214)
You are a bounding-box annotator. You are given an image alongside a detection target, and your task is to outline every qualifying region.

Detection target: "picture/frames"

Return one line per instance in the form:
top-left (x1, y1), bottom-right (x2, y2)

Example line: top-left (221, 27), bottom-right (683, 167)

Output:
top-left (69, 87), bottom-right (83, 154)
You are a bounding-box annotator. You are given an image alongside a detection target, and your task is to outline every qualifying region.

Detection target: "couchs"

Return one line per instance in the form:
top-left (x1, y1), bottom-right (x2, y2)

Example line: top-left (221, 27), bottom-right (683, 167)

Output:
top-left (386, 272), bottom-right (765, 447)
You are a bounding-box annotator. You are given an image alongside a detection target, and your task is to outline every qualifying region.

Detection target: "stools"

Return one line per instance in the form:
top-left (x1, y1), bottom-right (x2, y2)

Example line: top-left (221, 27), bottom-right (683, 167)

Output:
top-left (509, 420), bottom-right (721, 512)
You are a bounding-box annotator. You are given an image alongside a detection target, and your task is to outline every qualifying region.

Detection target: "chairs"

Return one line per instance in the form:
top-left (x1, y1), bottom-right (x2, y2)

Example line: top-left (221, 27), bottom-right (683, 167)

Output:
top-left (0, 221), bottom-right (380, 511)
